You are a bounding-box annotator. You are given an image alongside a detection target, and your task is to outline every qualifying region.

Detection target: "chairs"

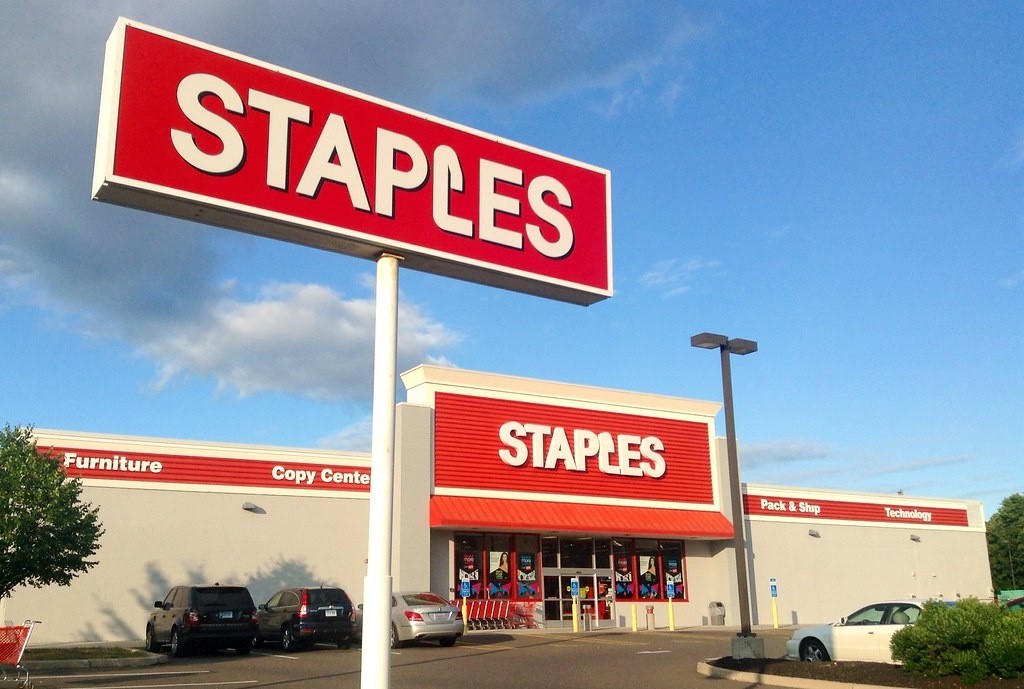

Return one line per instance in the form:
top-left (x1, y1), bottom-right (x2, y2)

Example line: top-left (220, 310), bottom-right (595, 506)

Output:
top-left (892, 611), bottom-right (910, 624)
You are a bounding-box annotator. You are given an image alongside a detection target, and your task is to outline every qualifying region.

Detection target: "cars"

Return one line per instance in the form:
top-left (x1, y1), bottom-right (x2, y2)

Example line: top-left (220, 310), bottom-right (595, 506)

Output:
top-left (354, 590), bottom-right (464, 648)
top-left (787, 598), bottom-right (931, 667)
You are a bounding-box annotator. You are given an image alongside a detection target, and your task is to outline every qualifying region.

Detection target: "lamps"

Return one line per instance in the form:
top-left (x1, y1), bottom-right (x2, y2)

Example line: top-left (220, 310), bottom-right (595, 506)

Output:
top-left (911, 535), bottom-right (920, 540)
top-left (243, 502), bottom-right (256, 510)
top-left (809, 530), bottom-right (818, 536)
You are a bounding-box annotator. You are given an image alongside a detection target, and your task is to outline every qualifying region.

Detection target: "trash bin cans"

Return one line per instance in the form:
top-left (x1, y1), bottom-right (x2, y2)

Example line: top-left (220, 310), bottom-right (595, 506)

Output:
top-left (710, 600), bottom-right (726, 626)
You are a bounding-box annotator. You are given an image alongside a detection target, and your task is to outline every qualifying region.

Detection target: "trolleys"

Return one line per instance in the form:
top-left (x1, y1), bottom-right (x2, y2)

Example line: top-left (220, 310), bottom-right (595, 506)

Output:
top-left (447, 597), bottom-right (541, 631)
top-left (0, 619), bottom-right (41, 689)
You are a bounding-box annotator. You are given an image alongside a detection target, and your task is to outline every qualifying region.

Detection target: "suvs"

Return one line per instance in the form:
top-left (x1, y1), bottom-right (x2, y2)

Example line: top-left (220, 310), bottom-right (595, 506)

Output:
top-left (146, 582), bottom-right (261, 658)
top-left (253, 586), bottom-right (357, 653)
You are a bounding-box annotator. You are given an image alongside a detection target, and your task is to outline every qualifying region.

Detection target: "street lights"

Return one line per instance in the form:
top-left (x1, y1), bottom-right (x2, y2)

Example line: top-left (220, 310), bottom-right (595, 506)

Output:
top-left (691, 331), bottom-right (766, 662)
top-left (1002, 539), bottom-right (1016, 591)
